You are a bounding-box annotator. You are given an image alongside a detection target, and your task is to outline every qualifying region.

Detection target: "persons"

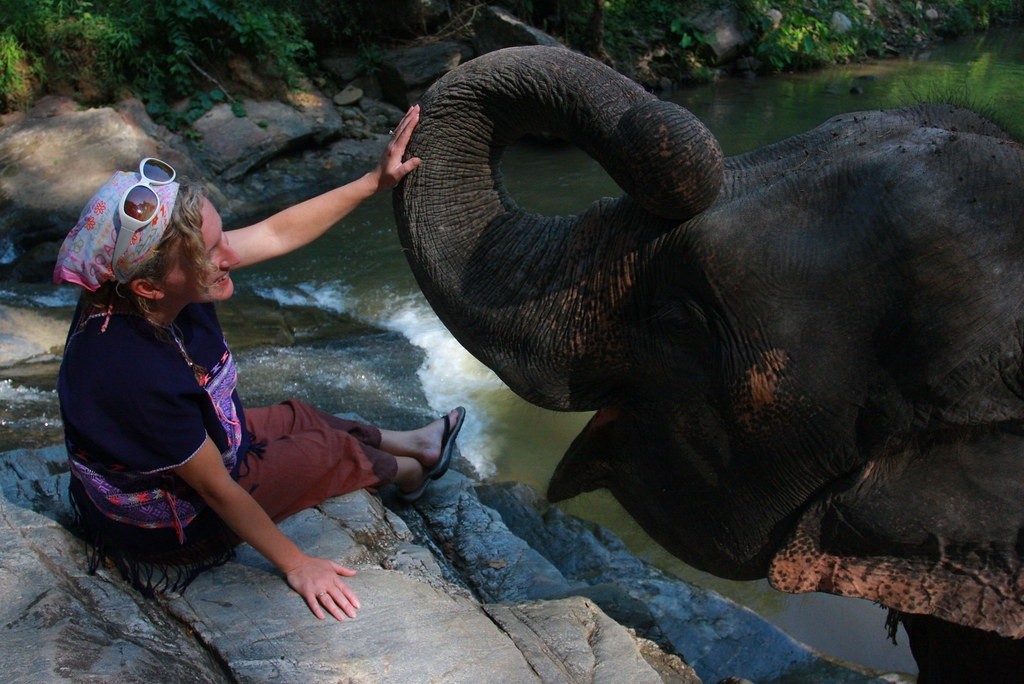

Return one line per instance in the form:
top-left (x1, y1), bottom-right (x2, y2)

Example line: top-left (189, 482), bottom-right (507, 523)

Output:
top-left (53, 105), bottom-right (465, 621)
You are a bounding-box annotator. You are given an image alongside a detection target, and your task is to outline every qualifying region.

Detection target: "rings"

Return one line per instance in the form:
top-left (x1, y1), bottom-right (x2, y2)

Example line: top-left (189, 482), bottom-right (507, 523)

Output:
top-left (318, 592), bottom-right (325, 599)
top-left (389, 130), bottom-right (395, 137)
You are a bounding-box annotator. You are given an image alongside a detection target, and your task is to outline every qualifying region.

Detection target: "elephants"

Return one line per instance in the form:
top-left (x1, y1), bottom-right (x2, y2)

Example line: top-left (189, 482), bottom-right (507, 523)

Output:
top-left (392, 44), bottom-right (1024, 683)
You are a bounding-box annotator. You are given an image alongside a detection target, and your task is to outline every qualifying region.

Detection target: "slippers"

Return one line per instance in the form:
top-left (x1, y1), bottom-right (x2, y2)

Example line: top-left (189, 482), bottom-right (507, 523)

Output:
top-left (426, 407), bottom-right (466, 480)
top-left (396, 477), bottom-right (432, 504)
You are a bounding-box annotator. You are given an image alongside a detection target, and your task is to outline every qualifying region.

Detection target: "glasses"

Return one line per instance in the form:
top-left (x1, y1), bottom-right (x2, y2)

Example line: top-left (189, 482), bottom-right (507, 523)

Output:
top-left (110, 157), bottom-right (177, 272)
